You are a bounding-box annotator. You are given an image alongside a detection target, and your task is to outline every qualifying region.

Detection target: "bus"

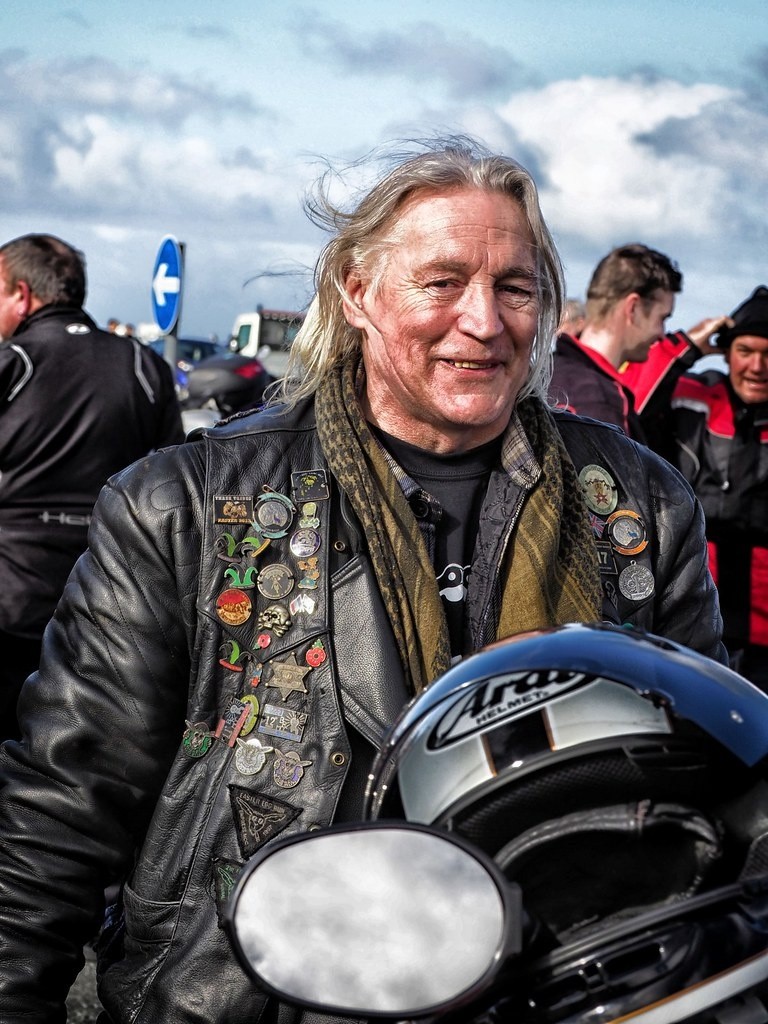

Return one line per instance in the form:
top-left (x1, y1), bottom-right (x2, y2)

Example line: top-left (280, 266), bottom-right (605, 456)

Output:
top-left (232, 311), bottom-right (304, 380)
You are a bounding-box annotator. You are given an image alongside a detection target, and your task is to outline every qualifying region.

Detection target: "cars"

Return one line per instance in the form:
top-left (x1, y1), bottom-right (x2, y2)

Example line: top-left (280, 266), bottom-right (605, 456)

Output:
top-left (150, 337), bottom-right (225, 392)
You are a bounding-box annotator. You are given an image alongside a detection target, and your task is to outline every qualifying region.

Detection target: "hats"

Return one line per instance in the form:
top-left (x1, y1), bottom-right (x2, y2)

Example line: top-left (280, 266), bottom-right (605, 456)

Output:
top-left (716, 285), bottom-right (768, 350)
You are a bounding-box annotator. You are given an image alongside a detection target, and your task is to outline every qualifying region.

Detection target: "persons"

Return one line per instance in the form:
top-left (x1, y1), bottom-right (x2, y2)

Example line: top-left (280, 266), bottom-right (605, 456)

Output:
top-left (0, 136), bottom-right (731, 1024)
top-left (0, 232), bottom-right (189, 731)
top-left (617, 284), bottom-right (768, 693)
top-left (546, 243), bottom-right (683, 438)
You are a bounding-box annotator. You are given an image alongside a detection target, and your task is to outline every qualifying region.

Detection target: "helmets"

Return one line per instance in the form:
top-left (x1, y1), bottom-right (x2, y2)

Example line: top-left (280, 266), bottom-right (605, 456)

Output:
top-left (358, 622), bottom-right (768, 1024)
top-left (186, 354), bottom-right (271, 420)
